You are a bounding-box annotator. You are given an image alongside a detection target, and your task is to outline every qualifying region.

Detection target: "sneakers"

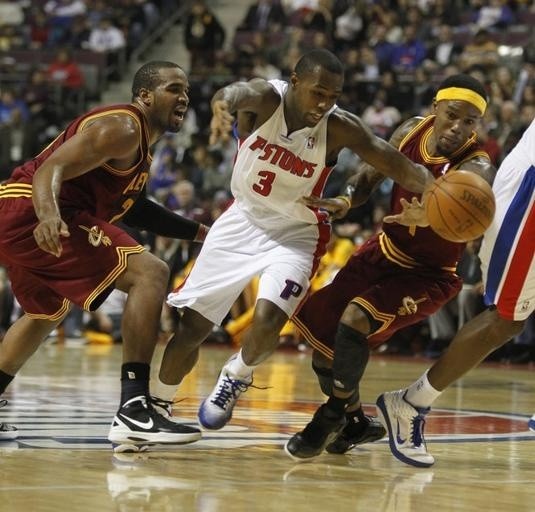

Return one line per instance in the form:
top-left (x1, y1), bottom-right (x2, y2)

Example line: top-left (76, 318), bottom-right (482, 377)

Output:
top-left (284, 402), bottom-right (346, 463)
top-left (282, 464), bottom-right (364, 489)
top-left (375, 386), bottom-right (434, 468)
top-left (108, 393), bottom-right (202, 444)
top-left (9, 308), bottom-right (123, 348)
top-left (0, 423), bottom-right (20, 440)
top-left (105, 470), bottom-right (201, 506)
top-left (379, 469), bottom-right (433, 511)
top-left (198, 353), bottom-right (253, 431)
top-left (325, 416), bottom-right (387, 454)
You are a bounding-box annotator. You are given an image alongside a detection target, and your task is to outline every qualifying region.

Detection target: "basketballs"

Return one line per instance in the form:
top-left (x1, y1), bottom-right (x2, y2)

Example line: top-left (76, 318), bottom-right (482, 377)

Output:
top-left (422, 172), bottom-right (495, 245)
